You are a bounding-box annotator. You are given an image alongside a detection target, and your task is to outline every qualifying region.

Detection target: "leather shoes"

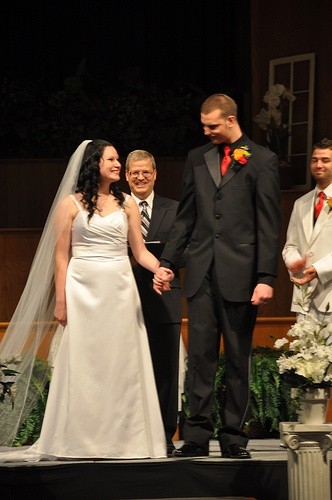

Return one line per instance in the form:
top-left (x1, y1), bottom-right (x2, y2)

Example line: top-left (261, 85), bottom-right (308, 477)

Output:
top-left (173, 443), bottom-right (209, 457)
top-left (221, 447), bottom-right (251, 459)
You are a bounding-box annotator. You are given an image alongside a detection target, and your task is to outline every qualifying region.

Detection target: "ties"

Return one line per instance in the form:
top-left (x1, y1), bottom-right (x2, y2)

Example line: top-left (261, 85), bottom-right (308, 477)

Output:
top-left (220, 146), bottom-right (232, 176)
top-left (139, 201), bottom-right (151, 240)
top-left (315, 191), bottom-right (326, 226)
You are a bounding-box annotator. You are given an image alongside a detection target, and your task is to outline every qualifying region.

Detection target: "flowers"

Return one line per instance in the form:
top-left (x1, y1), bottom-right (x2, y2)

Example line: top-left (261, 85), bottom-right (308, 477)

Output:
top-left (254, 82), bottom-right (296, 154)
top-left (326, 196), bottom-right (332, 210)
top-left (231, 144), bottom-right (251, 170)
top-left (274, 283), bottom-right (332, 391)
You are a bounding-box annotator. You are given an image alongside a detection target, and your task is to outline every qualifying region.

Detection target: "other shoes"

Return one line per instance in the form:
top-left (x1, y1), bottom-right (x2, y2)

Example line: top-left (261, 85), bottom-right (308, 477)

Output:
top-left (167, 440), bottom-right (176, 454)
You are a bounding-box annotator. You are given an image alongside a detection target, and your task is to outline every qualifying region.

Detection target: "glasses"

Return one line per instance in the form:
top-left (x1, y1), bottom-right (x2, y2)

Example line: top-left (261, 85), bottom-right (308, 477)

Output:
top-left (126, 170), bottom-right (155, 177)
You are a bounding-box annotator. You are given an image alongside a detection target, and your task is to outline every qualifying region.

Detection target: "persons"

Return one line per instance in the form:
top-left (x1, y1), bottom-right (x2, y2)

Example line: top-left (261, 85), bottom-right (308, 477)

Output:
top-left (153, 95), bottom-right (282, 458)
top-left (126, 150), bottom-right (191, 455)
top-left (0, 138), bottom-right (174, 463)
top-left (282, 138), bottom-right (332, 423)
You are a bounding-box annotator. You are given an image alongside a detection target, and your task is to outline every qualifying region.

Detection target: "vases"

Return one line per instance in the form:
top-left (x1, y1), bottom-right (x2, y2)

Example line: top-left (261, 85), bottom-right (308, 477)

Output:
top-left (276, 156), bottom-right (293, 190)
top-left (290, 388), bottom-right (329, 424)
